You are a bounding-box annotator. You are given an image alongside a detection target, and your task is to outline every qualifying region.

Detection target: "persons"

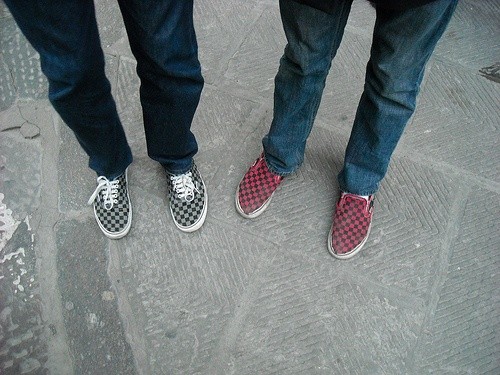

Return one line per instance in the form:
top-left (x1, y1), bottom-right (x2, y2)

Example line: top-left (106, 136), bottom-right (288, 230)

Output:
top-left (236, 0), bottom-right (458, 259)
top-left (4, 0), bottom-right (208, 240)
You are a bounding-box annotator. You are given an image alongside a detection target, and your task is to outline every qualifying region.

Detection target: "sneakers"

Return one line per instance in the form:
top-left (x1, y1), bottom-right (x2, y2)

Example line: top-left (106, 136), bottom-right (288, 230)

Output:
top-left (87, 167), bottom-right (132, 240)
top-left (235, 155), bottom-right (284, 219)
top-left (164, 160), bottom-right (208, 232)
top-left (327, 190), bottom-right (373, 259)
